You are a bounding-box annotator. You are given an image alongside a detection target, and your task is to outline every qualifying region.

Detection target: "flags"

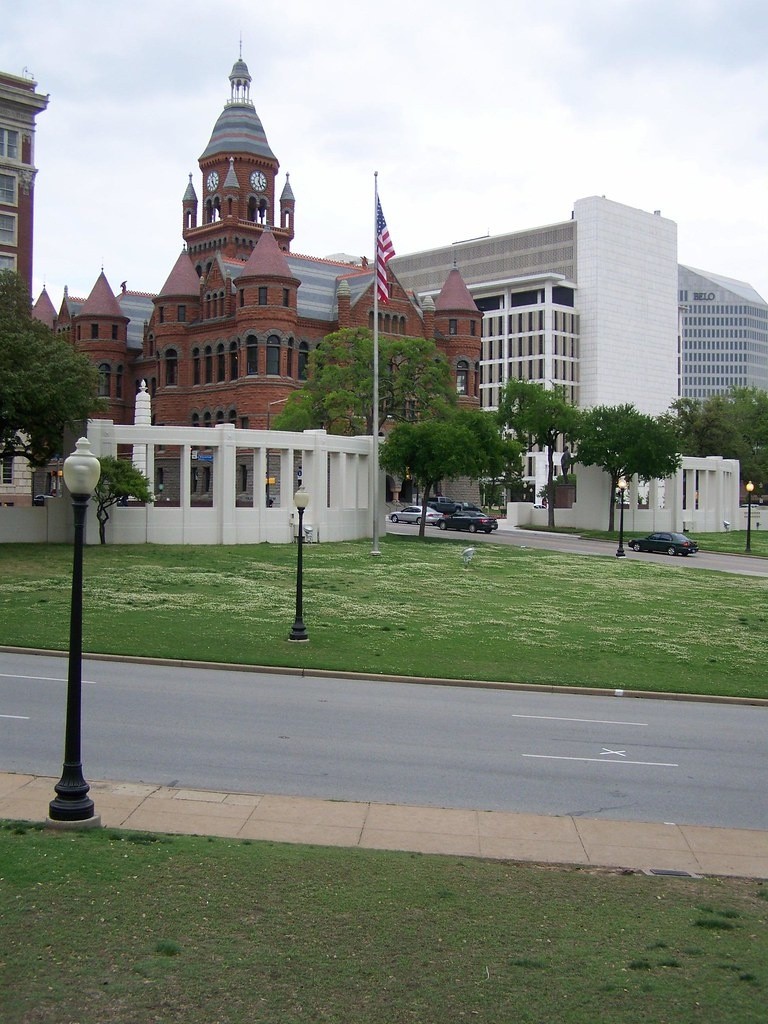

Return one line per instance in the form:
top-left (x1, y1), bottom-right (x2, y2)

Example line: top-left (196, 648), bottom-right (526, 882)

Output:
top-left (377, 192), bottom-right (397, 303)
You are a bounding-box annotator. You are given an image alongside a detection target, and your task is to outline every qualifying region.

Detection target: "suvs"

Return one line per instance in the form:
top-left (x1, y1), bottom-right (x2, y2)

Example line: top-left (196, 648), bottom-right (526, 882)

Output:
top-left (427, 496), bottom-right (463, 515)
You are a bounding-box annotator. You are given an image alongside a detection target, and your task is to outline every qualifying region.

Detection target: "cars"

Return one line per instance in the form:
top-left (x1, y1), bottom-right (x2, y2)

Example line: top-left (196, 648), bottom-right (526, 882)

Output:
top-left (389, 506), bottom-right (443, 525)
top-left (457, 501), bottom-right (483, 513)
top-left (435, 511), bottom-right (498, 533)
top-left (34, 495), bottom-right (54, 506)
top-left (628, 531), bottom-right (699, 557)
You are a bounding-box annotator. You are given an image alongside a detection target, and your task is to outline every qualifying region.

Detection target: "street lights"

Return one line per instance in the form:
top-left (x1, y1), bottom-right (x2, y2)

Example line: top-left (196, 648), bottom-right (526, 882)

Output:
top-left (48, 434), bottom-right (106, 819)
top-left (744, 480), bottom-right (755, 552)
top-left (289, 483), bottom-right (310, 640)
top-left (615, 476), bottom-right (629, 557)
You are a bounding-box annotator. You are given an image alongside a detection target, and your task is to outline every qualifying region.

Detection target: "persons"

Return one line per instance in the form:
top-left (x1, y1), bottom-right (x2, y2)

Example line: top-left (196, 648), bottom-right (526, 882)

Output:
top-left (562, 444), bottom-right (572, 485)
